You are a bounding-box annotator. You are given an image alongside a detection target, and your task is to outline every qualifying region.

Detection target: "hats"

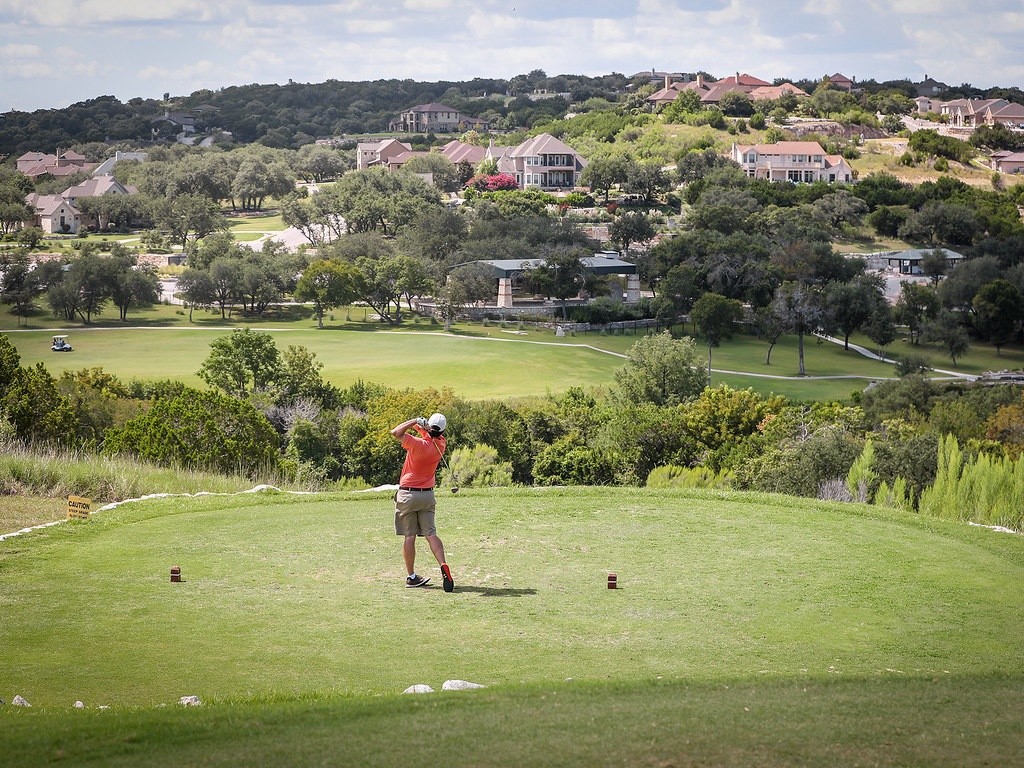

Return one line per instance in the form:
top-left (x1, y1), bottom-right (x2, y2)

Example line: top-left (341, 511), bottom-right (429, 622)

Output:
top-left (428, 413), bottom-right (446, 432)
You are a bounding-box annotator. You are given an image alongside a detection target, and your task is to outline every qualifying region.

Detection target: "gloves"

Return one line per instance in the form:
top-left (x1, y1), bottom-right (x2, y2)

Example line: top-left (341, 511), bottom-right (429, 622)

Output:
top-left (416, 418), bottom-right (427, 427)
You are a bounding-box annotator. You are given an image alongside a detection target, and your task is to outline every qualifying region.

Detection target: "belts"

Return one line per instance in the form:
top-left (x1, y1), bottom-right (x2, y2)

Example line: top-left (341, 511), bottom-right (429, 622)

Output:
top-left (399, 486), bottom-right (433, 491)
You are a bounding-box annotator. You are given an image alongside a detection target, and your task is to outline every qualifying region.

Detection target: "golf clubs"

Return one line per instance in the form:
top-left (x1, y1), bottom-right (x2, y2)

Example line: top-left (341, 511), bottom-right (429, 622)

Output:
top-left (424, 429), bottom-right (459, 494)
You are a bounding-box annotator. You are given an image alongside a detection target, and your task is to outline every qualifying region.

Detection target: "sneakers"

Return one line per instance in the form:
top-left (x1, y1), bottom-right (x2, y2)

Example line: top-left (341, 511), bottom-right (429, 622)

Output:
top-left (441, 563), bottom-right (454, 592)
top-left (406, 574), bottom-right (431, 588)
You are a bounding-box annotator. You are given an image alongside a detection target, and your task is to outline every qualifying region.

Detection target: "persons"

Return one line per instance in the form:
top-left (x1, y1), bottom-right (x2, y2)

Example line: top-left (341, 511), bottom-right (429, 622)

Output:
top-left (391, 413), bottom-right (455, 593)
